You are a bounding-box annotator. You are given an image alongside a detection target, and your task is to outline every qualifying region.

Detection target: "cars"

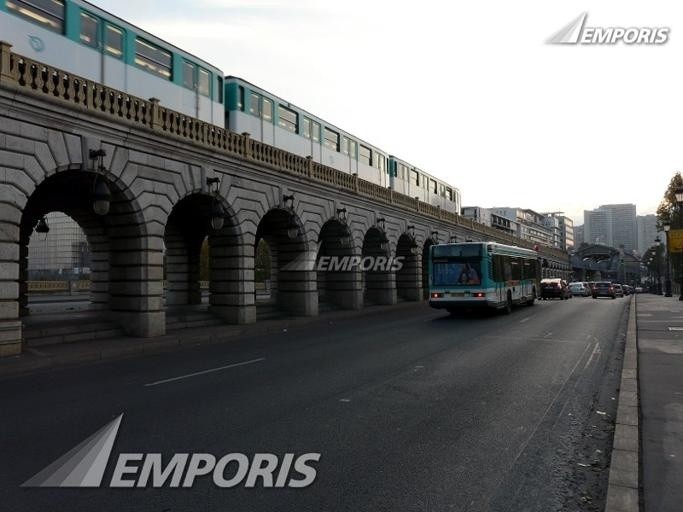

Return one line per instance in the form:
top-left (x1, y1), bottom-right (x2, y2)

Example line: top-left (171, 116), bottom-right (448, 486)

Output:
top-left (591, 281), bottom-right (635, 299)
top-left (637, 286), bottom-right (649, 292)
top-left (540, 278), bottom-right (592, 300)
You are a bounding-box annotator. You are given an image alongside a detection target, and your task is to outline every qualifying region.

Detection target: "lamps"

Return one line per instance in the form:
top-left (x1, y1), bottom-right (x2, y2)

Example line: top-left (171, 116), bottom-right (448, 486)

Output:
top-left (450, 236), bottom-right (457, 244)
top-left (206, 177), bottom-right (226, 232)
top-left (407, 225), bottom-right (417, 256)
top-left (89, 149), bottom-right (112, 215)
top-left (431, 231), bottom-right (439, 246)
top-left (35, 217), bottom-right (49, 241)
top-left (337, 208), bottom-right (351, 246)
top-left (377, 217), bottom-right (389, 252)
top-left (282, 195), bottom-right (300, 241)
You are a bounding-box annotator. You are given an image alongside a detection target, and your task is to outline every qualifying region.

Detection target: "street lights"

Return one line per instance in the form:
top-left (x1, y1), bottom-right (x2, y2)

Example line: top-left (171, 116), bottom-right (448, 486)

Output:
top-left (663, 220), bottom-right (673, 297)
top-left (674, 187), bottom-right (683, 300)
top-left (647, 236), bottom-right (663, 295)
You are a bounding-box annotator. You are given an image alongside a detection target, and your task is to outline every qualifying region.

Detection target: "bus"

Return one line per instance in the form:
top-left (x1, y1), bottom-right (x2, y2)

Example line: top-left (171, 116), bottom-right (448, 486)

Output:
top-left (426, 242), bottom-right (542, 317)
